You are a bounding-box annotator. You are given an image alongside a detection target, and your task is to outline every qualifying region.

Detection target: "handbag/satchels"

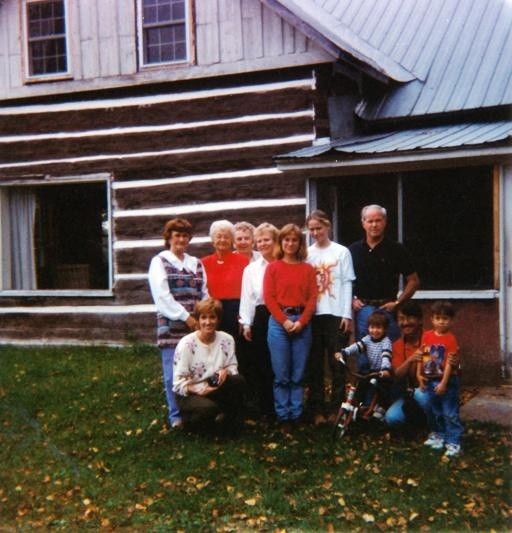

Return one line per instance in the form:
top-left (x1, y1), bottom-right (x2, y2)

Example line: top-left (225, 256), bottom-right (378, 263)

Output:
top-left (402, 391), bottom-right (426, 426)
top-left (169, 312), bottom-right (195, 332)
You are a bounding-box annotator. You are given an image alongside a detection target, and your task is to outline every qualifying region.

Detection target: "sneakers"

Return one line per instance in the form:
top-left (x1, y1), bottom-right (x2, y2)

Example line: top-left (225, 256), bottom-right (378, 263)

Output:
top-left (328, 409), bottom-right (338, 423)
top-left (445, 443), bottom-right (461, 456)
top-left (373, 405), bottom-right (386, 419)
top-left (314, 411), bottom-right (325, 427)
top-left (172, 418), bottom-right (185, 431)
top-left (424, 431), bottom-right (444, 449)
top-left (280, 419), bottom-right (291, 433)
top-left (294, 416), bottom-right (306, 430)
top-left (224, 412), bottom-right (241, 440)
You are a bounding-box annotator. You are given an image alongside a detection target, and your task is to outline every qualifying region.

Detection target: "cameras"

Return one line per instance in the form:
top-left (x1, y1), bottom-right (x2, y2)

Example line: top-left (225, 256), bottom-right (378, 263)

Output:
top-left (208, 373), bottom-right (219, 386)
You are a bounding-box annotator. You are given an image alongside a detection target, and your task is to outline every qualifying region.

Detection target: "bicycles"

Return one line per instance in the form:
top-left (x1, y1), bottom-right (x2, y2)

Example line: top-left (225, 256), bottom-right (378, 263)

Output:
top-left (331, 354), bottom-right (402, 441)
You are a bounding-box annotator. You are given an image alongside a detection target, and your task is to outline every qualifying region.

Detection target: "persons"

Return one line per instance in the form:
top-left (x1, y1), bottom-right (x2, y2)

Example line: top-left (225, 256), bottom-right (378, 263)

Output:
top-left (146, 218), bottom-right (210, 429)
top-left (232, 221), bottom-right (262, 260)
top-left (333, 309), bottom-right (393, 418)
top-left (262, 222), bottom-right (317, 436)
top-left (236, 221), bottom-right (276, 415)
top-left (171, 295), bottom-right (240, 434)
top-left (414, 299), bottom-right (463, 457)
top-left (300, 209), bottom-right (357, 426)
top-left (200, 218), bottom-right (248, 365)
top-left (382, 298), bottom-right (464, 432)
top-left (348, 203), bottom-right (422, 406)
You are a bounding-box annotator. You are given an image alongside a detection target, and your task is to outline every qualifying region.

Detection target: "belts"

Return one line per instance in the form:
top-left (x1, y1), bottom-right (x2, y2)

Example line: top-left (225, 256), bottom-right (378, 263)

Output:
top-left (283, 306), bottom-right (303, 315)
top-left (361, 298), bottom-right (394, 307)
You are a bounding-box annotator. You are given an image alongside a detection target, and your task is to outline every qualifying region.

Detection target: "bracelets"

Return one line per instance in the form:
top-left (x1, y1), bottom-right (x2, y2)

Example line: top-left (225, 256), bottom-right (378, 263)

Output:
top-left (457, 363), bottom-right (461, 369)
